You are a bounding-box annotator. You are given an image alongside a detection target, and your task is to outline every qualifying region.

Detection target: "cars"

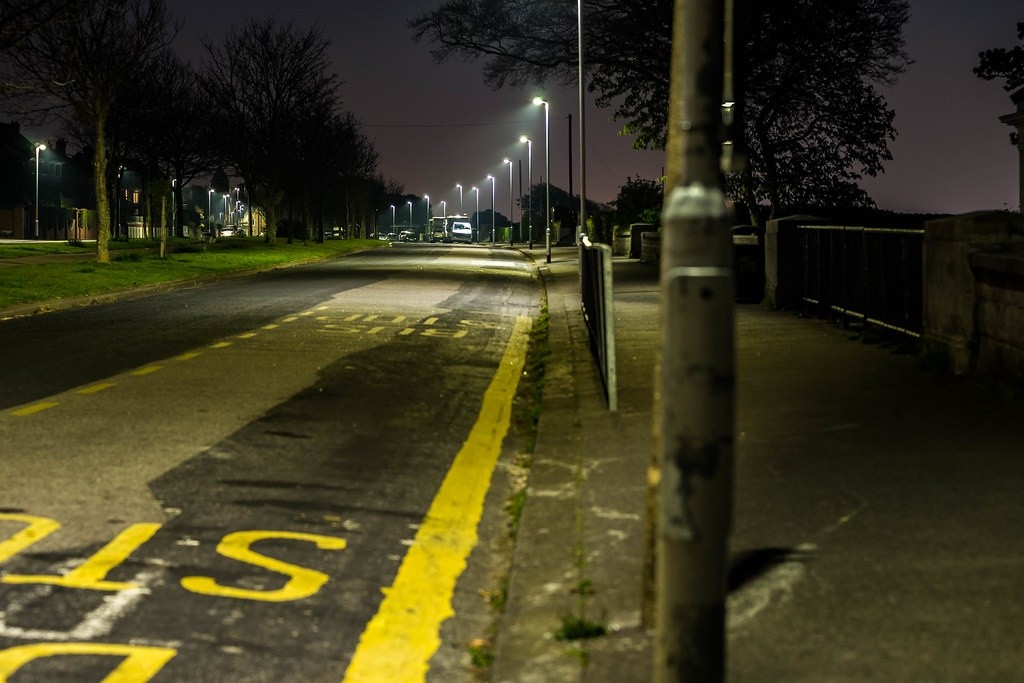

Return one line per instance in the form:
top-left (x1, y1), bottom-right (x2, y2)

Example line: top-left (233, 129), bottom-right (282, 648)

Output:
top-left (236, 228), bottom-right (247, 238)
top-left (370, 230), bottom-right (418, 243)
top-left (220, 226), bottom-right (238, 237)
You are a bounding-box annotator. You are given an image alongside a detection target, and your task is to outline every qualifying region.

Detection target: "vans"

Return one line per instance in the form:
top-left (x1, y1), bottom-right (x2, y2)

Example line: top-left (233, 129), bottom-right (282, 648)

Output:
top-left (449, 221), bottom-right (473, 244)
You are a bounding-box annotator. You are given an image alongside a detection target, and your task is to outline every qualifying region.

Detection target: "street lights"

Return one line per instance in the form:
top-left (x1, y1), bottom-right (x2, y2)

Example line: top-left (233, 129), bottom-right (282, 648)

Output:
top-left (504, 159), bottom-right (514, 246)
top-left (34, 144), bottom-right (46, 240)
top-left (208, 189), bottom-right (214, 232)
top-left (407, 201), bottom-right (412, 231)
top-left (391, 205), bottom-right (395, 233)
top-left (172, 179), bottom-right (177, 236)
top-left (456, 183), bottom-right (463, 216)
top-left (424, 194), bottom-right (431, 226)
top-left (520, 136), bottom-right (533, 250)
top-left (486, 175), bottom-right (496, 244)
top-left (472, 187), bottom-right (480, 243)
top-left (440, 201), bottom-right (446, 217)
top-left (533, 95), bottom-right (551, 263)
top-left (223, 187), bottom-right (244, 227)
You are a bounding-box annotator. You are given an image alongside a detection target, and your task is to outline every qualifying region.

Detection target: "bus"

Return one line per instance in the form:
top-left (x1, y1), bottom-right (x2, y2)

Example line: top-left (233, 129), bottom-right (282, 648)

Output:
top-left (426, 216), bottom-right (468, 242)
